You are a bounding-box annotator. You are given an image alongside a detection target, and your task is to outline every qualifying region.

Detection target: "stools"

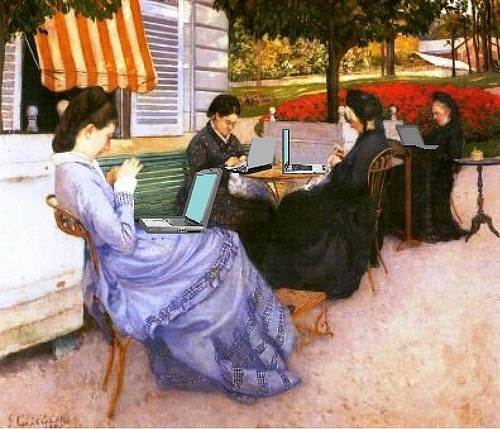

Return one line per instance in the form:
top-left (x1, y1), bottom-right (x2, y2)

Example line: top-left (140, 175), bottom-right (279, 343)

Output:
top-left (270, 287), bottom-right (335, 354)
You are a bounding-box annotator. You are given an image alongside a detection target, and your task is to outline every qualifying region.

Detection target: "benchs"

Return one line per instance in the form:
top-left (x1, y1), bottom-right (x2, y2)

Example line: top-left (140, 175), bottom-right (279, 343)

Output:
top-left (95, 142), bottom-right (277, 225)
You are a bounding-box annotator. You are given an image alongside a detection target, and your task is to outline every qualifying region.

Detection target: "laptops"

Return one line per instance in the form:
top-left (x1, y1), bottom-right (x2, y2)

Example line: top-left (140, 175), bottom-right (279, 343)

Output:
top-left (395, 124), bottom-right (441, 151)
top-left (281, 127), bottom-right (328, 176)
top-left (138, 164), bottom-right (224, 233)
top-left (224, 137), bottom-right (275, 173)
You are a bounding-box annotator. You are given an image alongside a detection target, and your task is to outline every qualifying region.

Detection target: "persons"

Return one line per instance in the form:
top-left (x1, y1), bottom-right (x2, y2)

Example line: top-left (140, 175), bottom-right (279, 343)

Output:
top-left (52, 85), bottom-right (302, 405)
top-left (248, 87), bottom-right (388, 299)
top-left (389, 91), bottom-right (470, 245)
top-left (174, 94), bottom-right (274, 271)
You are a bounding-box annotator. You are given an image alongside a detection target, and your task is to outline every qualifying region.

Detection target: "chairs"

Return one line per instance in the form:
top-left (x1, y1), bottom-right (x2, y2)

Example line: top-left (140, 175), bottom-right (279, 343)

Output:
top-left (47, 193), bottom-right (138, 421)
top-left (364, 146), bottom-right (394, 295)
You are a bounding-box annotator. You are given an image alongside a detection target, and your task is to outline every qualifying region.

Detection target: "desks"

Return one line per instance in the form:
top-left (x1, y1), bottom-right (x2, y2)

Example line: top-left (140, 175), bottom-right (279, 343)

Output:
top-left (239, 162), bottom-right (329, 206)
top-left (454, 157), bottom-right (499, 243)
